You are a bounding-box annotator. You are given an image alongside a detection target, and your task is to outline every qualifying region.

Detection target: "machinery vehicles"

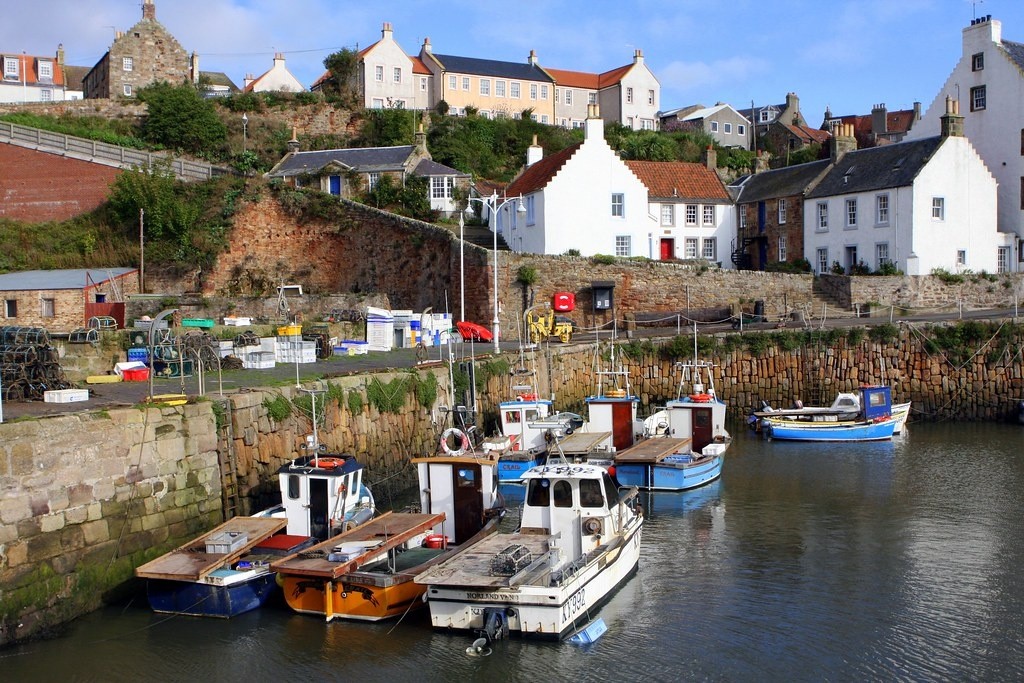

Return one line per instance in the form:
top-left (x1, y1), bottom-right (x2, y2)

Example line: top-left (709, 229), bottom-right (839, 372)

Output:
top-left (527, 306), bottom-right (573, 343)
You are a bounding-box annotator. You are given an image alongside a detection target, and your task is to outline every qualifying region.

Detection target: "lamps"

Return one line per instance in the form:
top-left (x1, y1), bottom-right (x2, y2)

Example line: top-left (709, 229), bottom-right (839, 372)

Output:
top-left (300, 443), bottom-right (307, 449)
top-left (457, 406), bottom-right (466, 410)
top-left (319, 443), bottom-right (327, 451)
top-left (306, 435), bottom-right (315, 450)
top-left (517, 396), bottom-right (523, 401)
top-left (438, 405), bottom-right (449, 411)
top-left (566, 428), bottom-right (573, 434)
top-left (466, 423), bottom-right (476, 433)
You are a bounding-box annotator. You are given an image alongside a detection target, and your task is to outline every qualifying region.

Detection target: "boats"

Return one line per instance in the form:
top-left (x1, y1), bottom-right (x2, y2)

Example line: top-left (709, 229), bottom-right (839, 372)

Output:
top-left (748, 357), bottom-right (912, 441)
top-left (135, 291), bottom-right (733, 658)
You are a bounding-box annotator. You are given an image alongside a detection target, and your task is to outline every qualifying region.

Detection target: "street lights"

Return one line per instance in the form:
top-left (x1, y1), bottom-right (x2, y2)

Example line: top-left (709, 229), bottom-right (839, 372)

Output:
top-left (242, 113), bottom-right (248, 153)
top-left (459, 212), bottom-right (464, 322)
top-left (464, 190), bottom-right (526, 354)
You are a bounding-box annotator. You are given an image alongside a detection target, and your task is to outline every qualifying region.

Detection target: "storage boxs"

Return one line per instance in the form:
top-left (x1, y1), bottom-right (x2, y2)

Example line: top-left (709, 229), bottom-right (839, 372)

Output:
top-left (182, 318), bottom-right (215, 327)
top-left (225, 317), bottom-right (251, 326)
top-left (205, 529), bottom-right (249, 553)
top-left (397, 529), bottom-right (433, 549)
top-left (335, 307), bottom-right (464, 358)
top-left (128, 348), bottom-right (150, 364)
top-left (122, 368), bottom-right (150, 382)
top-left (210, 325), bottom-right (316, 368)
top-left (44, 388), bottom-right (89, 404)
top-left (114, 360), bottom-right (148, 375)
top-left (238, 554), bottom-right (282, 567)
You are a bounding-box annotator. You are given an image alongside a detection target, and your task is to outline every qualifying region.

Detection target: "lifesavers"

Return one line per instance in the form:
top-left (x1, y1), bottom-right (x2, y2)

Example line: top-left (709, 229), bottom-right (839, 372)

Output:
top-left (515, 393), bottom-right (539, 402)
top-left (872, 415), bottom-right (891, 424)
top-left (688, 394), bottom-right (713, 403)
top-left (604, 390), bottom-right (625, 398)
top-left (309, 457), bottom-right (345, 470)
top-left (861, 384), bottom-right (876, 389)
top-left (440, 427), bottom-right (469, 457)
